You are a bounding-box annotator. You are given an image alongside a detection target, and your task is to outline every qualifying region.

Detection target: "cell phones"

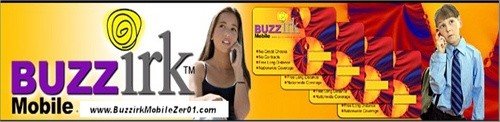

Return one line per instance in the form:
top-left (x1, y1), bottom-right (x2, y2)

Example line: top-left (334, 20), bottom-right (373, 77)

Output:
top-left (229, 50), bottom-right (235, 63)
top-left (436, 29), bottom-right (442, 37)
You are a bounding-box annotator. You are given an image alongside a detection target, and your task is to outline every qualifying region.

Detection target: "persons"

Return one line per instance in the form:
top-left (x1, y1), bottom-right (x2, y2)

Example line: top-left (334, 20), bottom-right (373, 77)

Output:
top-left (191, 7), bottom-right (252, 119)
top-left (425, 4), bottom-right (485, 119)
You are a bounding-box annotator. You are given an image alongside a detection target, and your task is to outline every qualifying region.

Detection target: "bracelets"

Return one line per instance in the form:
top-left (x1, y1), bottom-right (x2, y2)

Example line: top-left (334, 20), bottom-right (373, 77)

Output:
top-left (234, 81), bottom-right (246, 85)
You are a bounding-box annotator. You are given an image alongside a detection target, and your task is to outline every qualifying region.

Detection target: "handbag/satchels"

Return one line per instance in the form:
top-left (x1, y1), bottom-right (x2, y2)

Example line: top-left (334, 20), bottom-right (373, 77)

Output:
top-left (420, 46), bottom-right (447, 120)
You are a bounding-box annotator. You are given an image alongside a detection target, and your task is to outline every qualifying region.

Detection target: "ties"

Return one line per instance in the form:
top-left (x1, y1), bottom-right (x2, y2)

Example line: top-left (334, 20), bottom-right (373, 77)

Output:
top-left (450, 49), bottom-right (463, 115)
